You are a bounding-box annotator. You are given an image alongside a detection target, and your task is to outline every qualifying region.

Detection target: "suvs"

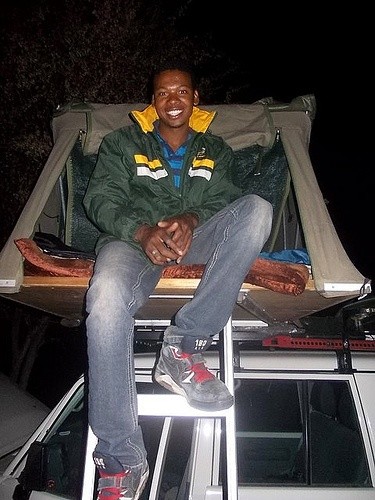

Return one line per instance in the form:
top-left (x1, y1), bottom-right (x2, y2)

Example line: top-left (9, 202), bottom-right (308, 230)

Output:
top-left (1, 326), bottom-right (374, 500)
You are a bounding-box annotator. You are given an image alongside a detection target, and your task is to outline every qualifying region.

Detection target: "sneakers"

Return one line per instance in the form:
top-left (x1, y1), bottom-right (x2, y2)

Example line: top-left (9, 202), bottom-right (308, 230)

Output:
top-left (155, 341), bottom-right (234, 411)
top-left (96, 458), bottom-right (149, 500)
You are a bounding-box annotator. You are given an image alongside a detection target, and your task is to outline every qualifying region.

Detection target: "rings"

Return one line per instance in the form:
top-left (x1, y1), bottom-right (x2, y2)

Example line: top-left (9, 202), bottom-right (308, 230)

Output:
top-left (151, 250), bottom-right (159, 256)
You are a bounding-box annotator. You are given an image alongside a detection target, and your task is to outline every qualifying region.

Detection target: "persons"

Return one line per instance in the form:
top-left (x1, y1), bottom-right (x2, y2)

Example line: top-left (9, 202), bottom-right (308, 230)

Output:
top-left (82, 58), bottom-right (275, 500)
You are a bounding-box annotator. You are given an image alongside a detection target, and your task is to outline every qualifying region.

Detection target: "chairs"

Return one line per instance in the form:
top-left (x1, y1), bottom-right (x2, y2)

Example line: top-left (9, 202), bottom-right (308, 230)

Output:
top-left (0, 93), bottom-right (373, 339)
